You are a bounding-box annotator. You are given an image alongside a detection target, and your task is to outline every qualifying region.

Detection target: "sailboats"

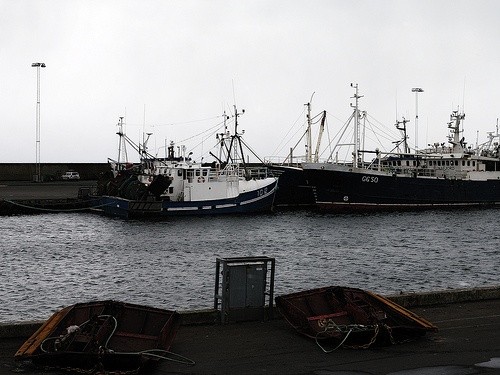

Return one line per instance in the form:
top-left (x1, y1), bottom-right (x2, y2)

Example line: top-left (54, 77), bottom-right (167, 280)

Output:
top-left (101, 83), bottom-right (500, 220)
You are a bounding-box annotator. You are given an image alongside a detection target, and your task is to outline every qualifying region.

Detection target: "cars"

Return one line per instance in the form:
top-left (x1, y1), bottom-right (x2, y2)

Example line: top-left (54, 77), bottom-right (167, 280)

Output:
top-left (61, 171), bottom-right (80, 181)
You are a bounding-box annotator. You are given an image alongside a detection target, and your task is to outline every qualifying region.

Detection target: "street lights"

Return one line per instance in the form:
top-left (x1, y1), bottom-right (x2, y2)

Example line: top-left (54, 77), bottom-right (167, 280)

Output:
top-left (31, 62), bottom-right (46, 183)
